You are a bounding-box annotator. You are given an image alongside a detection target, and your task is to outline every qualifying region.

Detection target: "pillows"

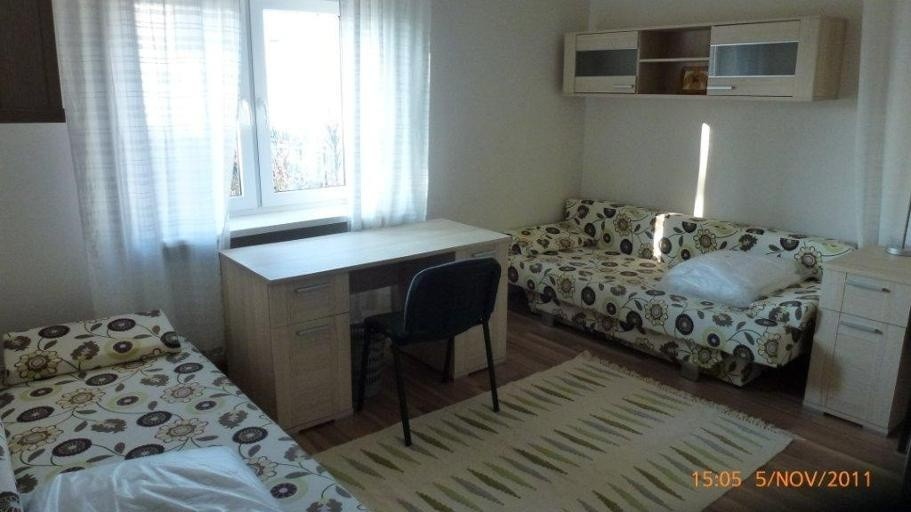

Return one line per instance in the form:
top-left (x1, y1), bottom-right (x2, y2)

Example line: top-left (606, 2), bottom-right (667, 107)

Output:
top-left (504, 216), bottom-right (595, 257)
top-left (658, 246), bottom-right (816, 312)
top-left (1, 306), bottom-right (185, 387)
top-left (1, 419), bottom-right (23, 512)
top-left (23, 445), bottom-right (287, 512)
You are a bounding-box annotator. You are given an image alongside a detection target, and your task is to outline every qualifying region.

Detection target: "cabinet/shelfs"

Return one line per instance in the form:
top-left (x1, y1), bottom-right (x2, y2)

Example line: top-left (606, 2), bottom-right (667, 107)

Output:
top-left (215, 216), bottom-right (514, 440)
top-left (562, 28), bottom-right (637, 99)
top-left (803, 239), bottom-right (910, 441)
top-left (635, 22), bottom-right (712, 100)
top-left (706, 13), bottom-right (852, 103)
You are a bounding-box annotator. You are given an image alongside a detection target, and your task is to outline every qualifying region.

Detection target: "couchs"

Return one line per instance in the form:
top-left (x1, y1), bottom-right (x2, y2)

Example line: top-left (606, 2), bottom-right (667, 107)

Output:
top-left (498, 196), bottom-right (861, 389)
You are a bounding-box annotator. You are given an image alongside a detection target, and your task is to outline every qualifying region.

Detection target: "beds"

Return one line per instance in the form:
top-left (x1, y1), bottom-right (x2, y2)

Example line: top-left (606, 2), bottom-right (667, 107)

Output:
top-left (1, 302), bottom-right (374, 512)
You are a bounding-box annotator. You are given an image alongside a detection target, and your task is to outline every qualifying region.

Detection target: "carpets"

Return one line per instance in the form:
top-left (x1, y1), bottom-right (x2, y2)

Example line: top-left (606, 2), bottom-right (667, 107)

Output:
top-left (301, 347), bottom-right (801, 510)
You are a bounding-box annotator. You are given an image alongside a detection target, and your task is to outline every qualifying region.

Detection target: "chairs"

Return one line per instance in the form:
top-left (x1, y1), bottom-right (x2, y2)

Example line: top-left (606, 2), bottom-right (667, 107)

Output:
top-left (354, 252), bottom-right (504, 447)
